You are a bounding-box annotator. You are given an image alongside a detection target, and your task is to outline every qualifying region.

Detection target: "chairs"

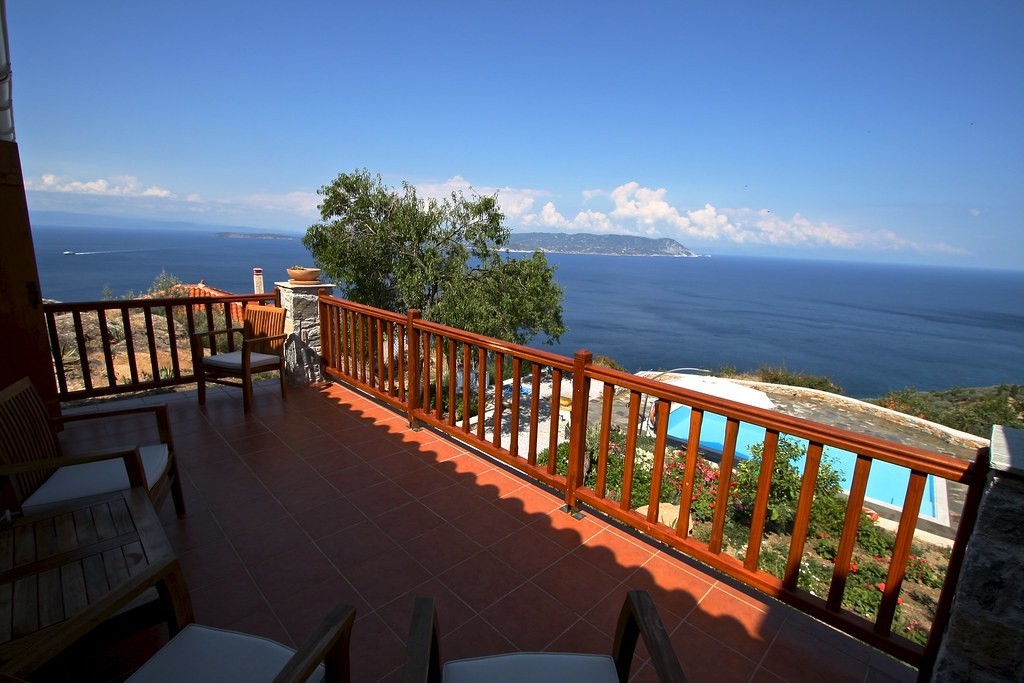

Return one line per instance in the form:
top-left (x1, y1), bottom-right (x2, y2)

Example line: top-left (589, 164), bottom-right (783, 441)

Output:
top-left (0, 375), bottom-right (186, 521)
top-left (401, 589), bottom-right (688, 683)
top-left (189, 304), bottom-right (289, 413)
top-left (0, 554), bottom-right (360, 683)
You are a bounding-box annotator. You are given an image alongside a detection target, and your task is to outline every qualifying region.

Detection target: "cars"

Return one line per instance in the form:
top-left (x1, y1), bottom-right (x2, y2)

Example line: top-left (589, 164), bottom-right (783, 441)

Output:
top-left (393, 334), bottom-right (424, 360)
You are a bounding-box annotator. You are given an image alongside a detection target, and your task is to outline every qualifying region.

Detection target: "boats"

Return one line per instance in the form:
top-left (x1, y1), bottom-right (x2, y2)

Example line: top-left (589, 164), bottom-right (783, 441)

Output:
top-left (64, 250), bottom-right (75, 255)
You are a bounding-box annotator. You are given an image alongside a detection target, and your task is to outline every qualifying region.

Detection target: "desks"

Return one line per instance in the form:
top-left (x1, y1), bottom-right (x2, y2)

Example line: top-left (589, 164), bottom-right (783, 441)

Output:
top-left (0, 485), bottom-right (197, 682)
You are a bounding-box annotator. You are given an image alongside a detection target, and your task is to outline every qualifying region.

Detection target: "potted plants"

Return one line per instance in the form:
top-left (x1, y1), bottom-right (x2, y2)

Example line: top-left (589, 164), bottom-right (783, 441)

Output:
top-left (286, 265), bottom-right (321, 285)
